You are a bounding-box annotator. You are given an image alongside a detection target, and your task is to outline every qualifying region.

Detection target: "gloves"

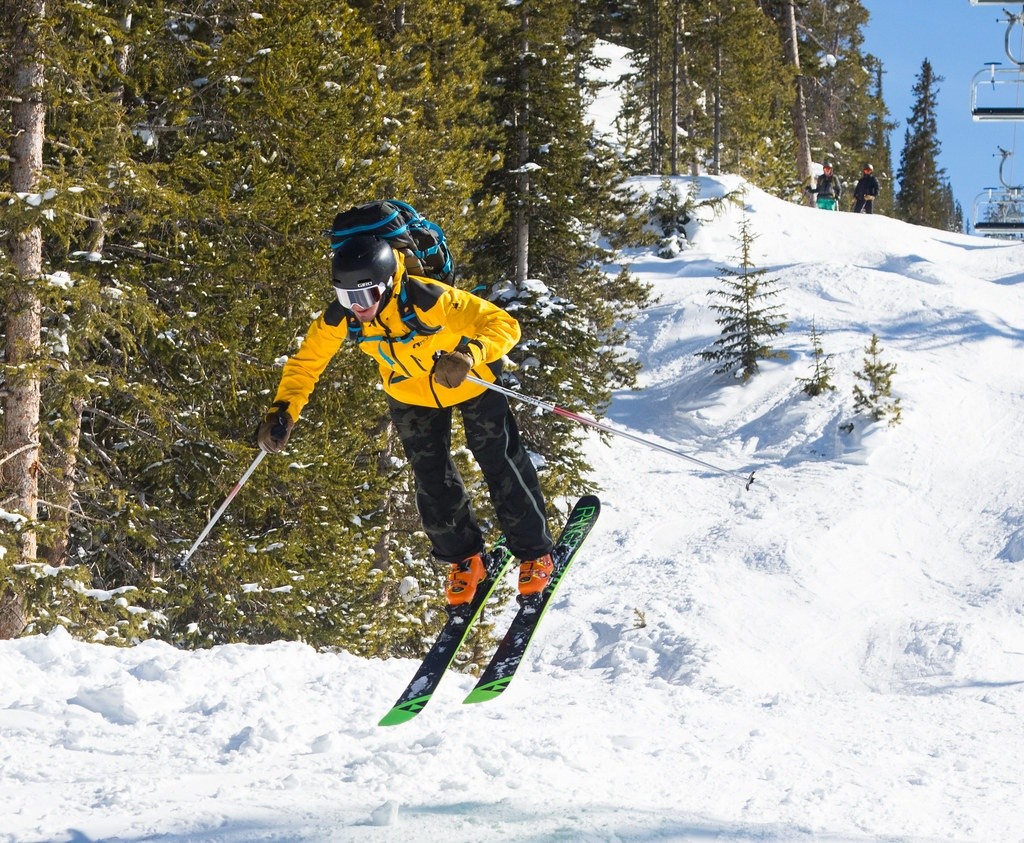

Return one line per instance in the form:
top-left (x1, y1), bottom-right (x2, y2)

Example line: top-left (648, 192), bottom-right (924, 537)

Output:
top-left (805, 186), bottom-right (812, 194)
top-left (429, 345), bottom-right (475, 389)
top-left (835, 193), bottom-right (841, 200)
top-left (254, 401), bottom-right (294, 456)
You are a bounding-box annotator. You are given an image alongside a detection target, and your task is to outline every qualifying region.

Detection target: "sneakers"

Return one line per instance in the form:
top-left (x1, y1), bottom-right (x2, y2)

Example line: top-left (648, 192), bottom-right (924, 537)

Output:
top-left (446, 550), bottom-right (487, 605)
top-left (518, 553), bottom-right (554, 595)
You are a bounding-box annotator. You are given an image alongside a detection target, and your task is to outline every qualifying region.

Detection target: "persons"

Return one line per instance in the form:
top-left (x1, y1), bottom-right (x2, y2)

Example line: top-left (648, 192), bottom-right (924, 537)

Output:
top-left (257, 236), bottom-right (553, 605)
top-left (805, 163), bottom-right (840, 210)
top-left (854, 164), bottom-right (879, 213)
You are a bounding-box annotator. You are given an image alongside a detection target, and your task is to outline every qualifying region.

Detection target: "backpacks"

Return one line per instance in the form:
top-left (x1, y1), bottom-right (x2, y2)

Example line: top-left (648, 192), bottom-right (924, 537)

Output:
top-left (329, 199), bottom-right (457, 348)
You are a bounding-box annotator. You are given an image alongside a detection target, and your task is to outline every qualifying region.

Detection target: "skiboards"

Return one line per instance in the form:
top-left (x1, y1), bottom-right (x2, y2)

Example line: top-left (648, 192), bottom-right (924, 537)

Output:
top-left (375, 492), bottom-right (603, 730)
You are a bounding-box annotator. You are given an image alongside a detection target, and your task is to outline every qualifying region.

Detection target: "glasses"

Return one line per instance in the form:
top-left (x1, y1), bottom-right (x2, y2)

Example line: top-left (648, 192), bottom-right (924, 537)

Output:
top-left (333, 284), bottom-right (380, 309)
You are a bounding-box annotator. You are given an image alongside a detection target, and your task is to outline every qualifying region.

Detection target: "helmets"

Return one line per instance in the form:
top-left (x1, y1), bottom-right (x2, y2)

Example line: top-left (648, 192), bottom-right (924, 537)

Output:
top-left (332, 237), bottom-right (395, 292)
top-left (863, 164), bottom-right (873, 172)
top-left (823, 162), bottom-right (833, 169)
